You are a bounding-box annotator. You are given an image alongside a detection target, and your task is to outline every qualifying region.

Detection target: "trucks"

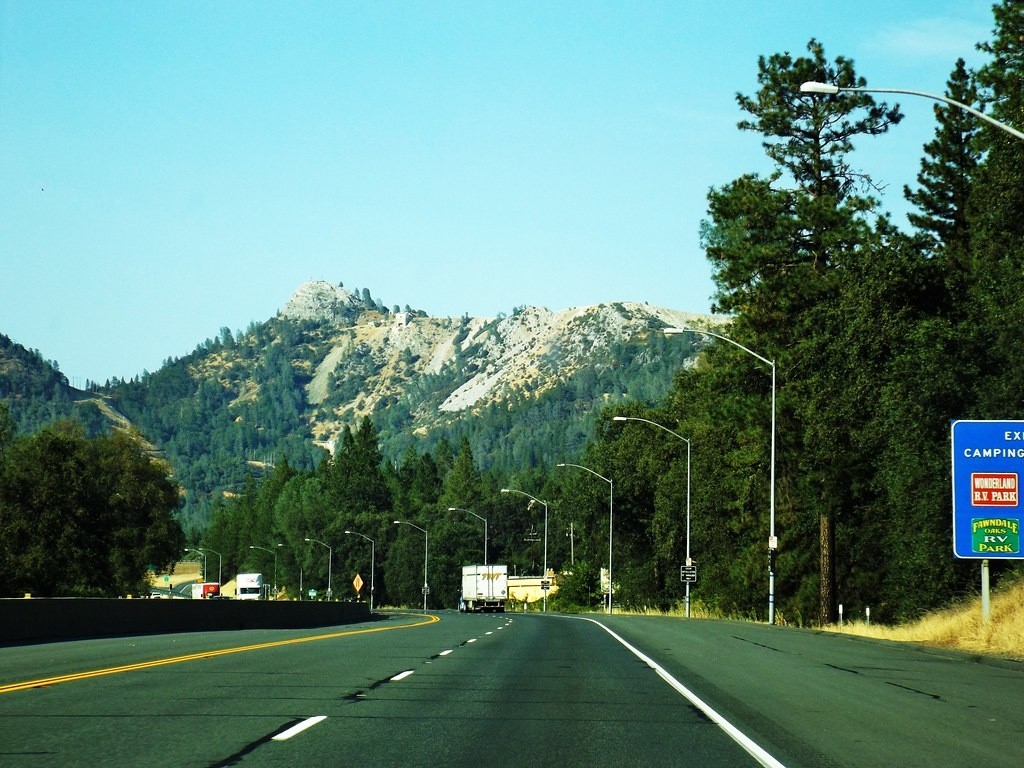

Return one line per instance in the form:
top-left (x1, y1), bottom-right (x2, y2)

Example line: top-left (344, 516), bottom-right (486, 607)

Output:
top-left (150, 592), bottom-right (161, 599)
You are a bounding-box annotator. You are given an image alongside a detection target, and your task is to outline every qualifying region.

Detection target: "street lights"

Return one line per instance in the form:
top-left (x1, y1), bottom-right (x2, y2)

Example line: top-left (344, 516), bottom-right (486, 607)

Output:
top-left (613, 417), bottom-right (690, 618)
top-left (278, 544), bottom-right (303, 601)
top-left (500, 489), bottom-right (547, 613)
top-left (663, 327), bottom-right (776, 624)
top-left (394, 521), bottom-right (427, 611)
top-left (448, 508), bottom-right (487, 565)
top-left (305, 539), bottom-right (331, 601)
top-left (185, 549), bottom-right (207, 582)
top-left (557, 464), bottom-right (613, 616)
top-left (199, 548), bottom-right (221, 586)
top-left (249, 546), bottom-right (277, 599)
top-left (345, 530), bottom-right (374, 611)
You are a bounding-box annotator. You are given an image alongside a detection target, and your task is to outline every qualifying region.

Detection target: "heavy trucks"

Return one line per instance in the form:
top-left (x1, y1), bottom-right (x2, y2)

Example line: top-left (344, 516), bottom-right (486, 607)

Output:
top-left (235, 574), bottom-right (263, 600)
top-left (457, 566), bottom-right (507, 614)
top-left (191, 582), bottom-right (219, 599)
